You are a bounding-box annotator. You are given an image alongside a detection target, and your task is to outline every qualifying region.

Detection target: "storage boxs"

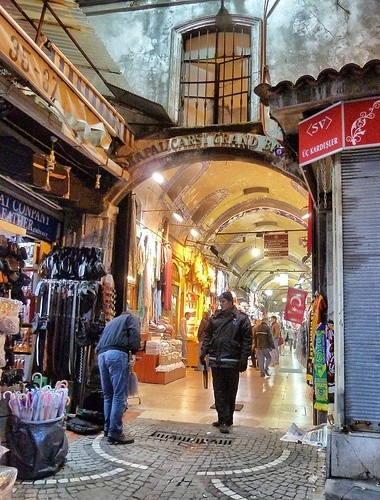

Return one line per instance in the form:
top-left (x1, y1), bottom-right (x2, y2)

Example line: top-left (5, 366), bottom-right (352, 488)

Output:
top-left (0, 464), bottom-right (18, 500)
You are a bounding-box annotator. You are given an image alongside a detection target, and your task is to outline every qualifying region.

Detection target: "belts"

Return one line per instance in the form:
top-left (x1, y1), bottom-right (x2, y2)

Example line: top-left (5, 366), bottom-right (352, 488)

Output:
top-left (33, 280), bottom-right (88, 380)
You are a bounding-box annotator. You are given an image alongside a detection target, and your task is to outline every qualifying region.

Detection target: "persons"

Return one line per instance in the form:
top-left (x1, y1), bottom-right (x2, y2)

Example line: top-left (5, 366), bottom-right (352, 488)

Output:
top-left (199, 291), bottom-right (253, 433)
top-left (280, 326), bottom-right (297, 349)
top-left (95, 312), bottom-right (141, 443)
top-left (271, 316), bottom-right (280, 366)
top-left (253, 319), bottom-right (275, 377)
top-left (250, 319), bottom-right (261, 367)
top-left (194, 312), bottom-right (210, 371)
top-left (178, 312), bottom-right (191, 364)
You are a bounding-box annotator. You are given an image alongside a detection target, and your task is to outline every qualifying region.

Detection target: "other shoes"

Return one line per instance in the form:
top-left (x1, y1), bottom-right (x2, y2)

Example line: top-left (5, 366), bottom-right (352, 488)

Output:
top-left (263, 369), bottom-right (269, 376)
top-left (213, 421), bottom-right (220, 427)
top-left (220, 423), bottom-right (229, 433)
top-left (249, 364), bottom-right (256, 368)
top-left (260, 373), bottom-right (265, 377)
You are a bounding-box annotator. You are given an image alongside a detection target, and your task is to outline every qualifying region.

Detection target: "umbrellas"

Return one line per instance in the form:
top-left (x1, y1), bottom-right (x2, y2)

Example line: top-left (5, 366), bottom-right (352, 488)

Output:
top-left (3, 373), bottom-right (71, 421)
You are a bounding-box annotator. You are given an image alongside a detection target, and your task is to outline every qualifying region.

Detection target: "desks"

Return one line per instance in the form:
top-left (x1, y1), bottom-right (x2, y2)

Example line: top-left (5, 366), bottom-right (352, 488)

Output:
top-left (185, 340), bottom-right (199, 367)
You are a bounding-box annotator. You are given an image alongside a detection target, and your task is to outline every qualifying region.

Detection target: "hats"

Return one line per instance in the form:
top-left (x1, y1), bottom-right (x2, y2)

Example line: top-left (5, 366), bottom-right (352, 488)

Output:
top-left (219, 291), bottom-right (233, 301)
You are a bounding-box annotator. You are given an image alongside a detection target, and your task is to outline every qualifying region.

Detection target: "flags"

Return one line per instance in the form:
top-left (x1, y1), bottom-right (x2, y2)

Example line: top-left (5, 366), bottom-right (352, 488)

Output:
top-left (284, 287), bottom-right (308, 324)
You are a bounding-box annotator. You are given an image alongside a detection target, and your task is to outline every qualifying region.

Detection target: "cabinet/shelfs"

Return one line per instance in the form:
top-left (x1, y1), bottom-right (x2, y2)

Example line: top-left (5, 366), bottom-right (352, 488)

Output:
top-left (132, 351), bottom-right (186, 385)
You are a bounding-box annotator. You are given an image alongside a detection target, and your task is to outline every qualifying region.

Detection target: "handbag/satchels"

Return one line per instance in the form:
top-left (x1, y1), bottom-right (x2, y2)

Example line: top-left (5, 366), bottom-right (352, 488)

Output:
top-left (37, 246), bottom-right (107, 280)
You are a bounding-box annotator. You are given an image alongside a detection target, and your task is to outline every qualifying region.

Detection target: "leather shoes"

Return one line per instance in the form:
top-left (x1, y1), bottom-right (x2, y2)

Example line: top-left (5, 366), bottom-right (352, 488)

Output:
top-left (103, 431), bottom-right (108, 436)
top-left (108, 435), bottom-right (134, 445)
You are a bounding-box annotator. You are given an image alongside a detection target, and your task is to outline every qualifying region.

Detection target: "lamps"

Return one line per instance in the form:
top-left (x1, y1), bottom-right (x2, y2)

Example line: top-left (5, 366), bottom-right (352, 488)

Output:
top-left (297, 237), bottom-right (308, 248)
top-left (203, 254), bottom-right (229, 268)
top-left (185, 239), bottom-right (219, 258)
top-left (104, 135), bottom-right (125, 159)
top-left (139, 208), bottom-right (184, 222)
top-left (166, 223), bottom-right (200, 237)
